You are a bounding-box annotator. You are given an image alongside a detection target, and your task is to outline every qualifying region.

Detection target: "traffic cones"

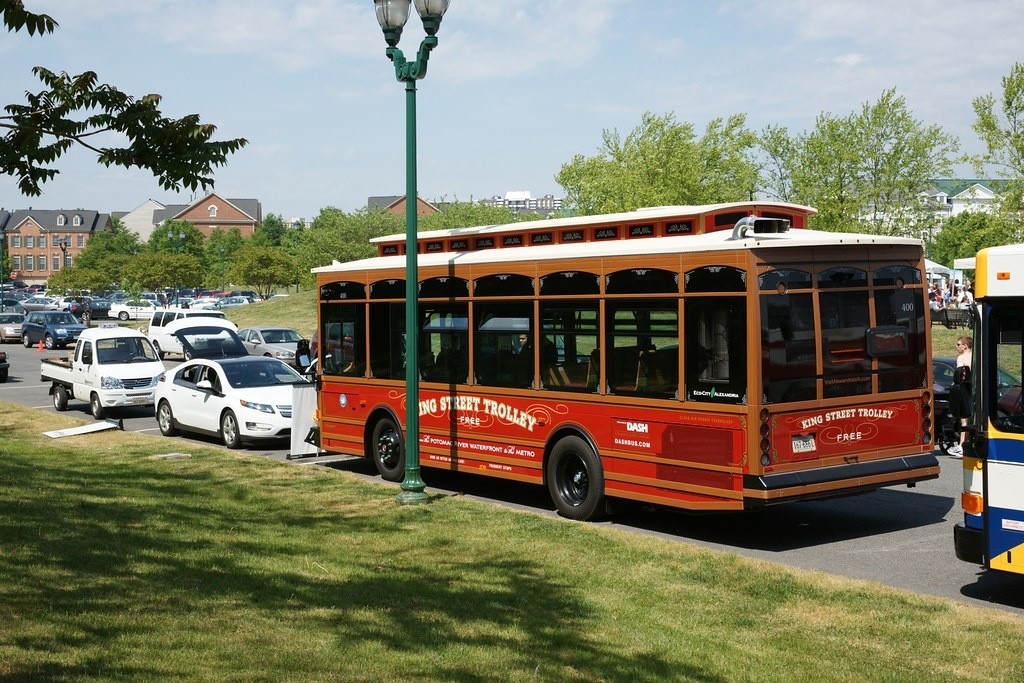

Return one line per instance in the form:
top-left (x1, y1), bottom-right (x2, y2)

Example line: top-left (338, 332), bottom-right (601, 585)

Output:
top-left (38, 340), bottom-right (46, 352)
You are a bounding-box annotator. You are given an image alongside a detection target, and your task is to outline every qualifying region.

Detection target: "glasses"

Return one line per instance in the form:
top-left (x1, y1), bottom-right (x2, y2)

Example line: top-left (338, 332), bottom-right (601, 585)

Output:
top-left (957, 343), bottom-right (964, 346)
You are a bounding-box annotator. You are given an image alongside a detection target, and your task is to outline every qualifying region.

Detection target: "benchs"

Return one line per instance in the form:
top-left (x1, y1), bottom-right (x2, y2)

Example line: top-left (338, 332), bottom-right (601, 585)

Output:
top-left (929, 308), bottom-right (971, 330)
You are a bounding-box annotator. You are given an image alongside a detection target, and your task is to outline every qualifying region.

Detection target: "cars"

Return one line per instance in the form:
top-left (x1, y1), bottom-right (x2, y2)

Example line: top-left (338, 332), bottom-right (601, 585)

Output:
top-left (151, 317), bottom-right (317, 448)
top-left (0, 352), bottom-right (10, 383)
top-left (0, 311), bottom-right (28, 343)
top-left (930, 356), bottom-right (1022, 455)
top-left (0, 280), bottom-right (290, 321)
top-left (306, 320), bottom-right (353, 371)
top-left (235, 326), bottom-right (308, 366)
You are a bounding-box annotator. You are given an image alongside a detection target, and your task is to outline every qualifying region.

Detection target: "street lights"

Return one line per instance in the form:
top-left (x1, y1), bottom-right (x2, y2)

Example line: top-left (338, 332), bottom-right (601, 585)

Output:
top-left (168, 229), bottom-right (185, 307)
top-left (219, 245), bottom-right (227, 293)
top-left (56, 235), bottom-right (69, 292)
top-left (371, 0), bottom-right (453, 502)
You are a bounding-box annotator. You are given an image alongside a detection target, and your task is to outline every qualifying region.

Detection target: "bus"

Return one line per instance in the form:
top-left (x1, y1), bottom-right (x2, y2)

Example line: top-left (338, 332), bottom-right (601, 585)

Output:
top-left (948, 243), bottom-right (1024, 577)
top-left (297, 201), bottom-right (942, 521)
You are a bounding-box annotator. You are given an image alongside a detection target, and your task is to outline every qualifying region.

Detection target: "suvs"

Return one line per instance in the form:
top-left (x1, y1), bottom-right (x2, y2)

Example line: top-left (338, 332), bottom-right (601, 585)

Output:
top-left (21, 310), bottom-right (90, 350)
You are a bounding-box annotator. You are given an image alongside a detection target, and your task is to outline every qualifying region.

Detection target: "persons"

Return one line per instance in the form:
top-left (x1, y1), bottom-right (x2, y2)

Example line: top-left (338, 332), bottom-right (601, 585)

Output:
top-left (946, 337), bottom-right (973, 459)
top-left (928, 276), bottom-right (976, 329)
top-left (518, 335), bottom-right (529, 353)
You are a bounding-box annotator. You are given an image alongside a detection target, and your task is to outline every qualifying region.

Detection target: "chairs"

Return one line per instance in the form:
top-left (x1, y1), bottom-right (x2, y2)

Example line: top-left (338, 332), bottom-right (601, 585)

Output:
top-left (577, 342), bottom-right (676, 392)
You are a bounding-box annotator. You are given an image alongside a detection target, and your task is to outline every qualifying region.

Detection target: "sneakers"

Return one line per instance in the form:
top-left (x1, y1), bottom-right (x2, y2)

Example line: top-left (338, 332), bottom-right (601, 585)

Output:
top-left (947, 445), bottom-right (964, 457)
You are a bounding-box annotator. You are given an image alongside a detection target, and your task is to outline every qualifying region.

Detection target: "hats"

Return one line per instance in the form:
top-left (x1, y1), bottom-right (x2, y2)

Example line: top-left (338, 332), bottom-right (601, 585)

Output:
top-left (929, 293), bottom-right (937, 298)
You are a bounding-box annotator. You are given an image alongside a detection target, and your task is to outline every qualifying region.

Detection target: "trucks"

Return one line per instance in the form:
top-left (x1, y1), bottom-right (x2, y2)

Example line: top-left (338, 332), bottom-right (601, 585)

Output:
top-left (40, 322), bottom-right (166, 418)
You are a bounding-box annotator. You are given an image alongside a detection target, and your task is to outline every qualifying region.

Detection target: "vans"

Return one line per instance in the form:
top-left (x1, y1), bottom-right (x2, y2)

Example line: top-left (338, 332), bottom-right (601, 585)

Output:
top-left (146, 307), bottom-right (238, 360)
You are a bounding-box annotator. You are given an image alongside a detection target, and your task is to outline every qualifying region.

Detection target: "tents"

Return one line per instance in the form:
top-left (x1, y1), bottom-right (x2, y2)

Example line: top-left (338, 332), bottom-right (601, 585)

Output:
top-left (925, 258), bottom-right (976, 294)
